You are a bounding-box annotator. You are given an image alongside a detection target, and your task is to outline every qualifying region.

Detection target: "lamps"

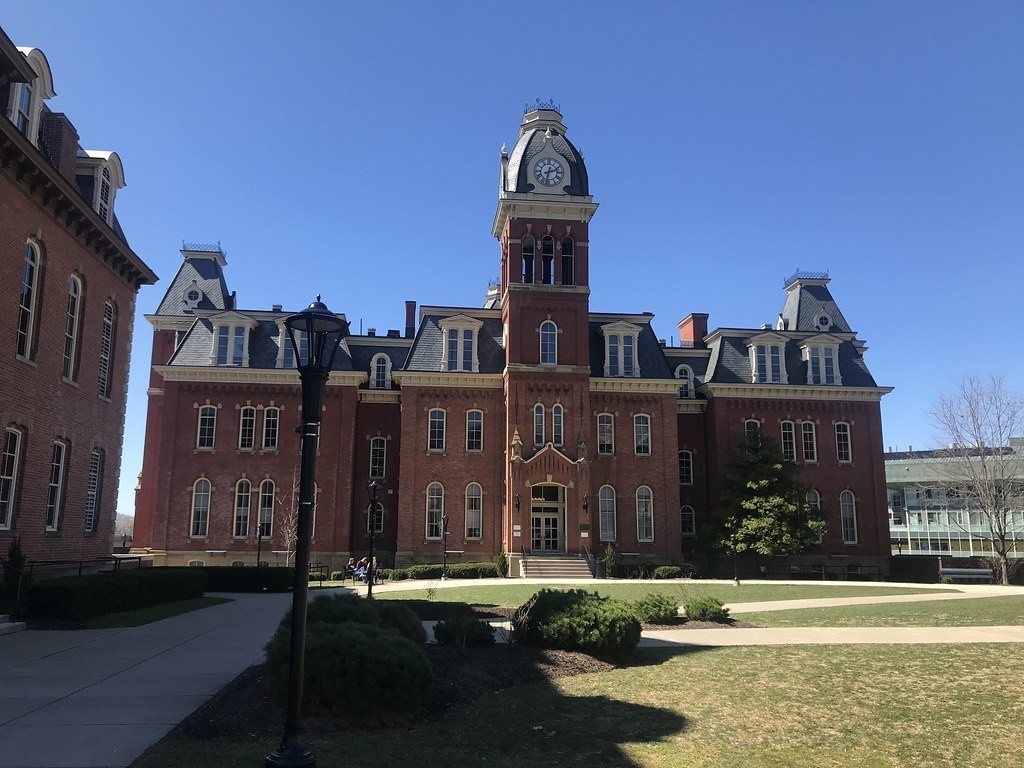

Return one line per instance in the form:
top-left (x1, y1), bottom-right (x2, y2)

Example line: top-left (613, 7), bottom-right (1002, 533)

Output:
top-left (583, 494), bottom-right (590, 514)
top-left (514, 493), bottom-right (522, 513)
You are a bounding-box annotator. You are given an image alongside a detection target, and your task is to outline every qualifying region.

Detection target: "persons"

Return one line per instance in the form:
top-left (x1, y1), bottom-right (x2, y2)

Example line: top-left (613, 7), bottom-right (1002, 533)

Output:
top-left (346, 557), bottom-right (381, 586)
top-left (121, 534), bottom-right (128, 547)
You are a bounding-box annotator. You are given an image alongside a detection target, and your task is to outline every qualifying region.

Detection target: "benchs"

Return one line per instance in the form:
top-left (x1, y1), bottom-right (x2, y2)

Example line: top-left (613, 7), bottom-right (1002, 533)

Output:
top-left (939, 557), bottom-right (994, 583)
top-left (308, 562), bottom-right (329, 580)
top-left (341, 566), bottom-right (383, 586)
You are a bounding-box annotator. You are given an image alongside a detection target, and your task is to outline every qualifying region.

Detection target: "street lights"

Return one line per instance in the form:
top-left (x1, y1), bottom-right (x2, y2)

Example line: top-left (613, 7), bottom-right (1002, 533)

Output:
top-left (260, 293), bottom-right (352, 767)
top-left (365, 480), bottom-right (383, 603)
top-left (440, 514), bottom-right (450, 580)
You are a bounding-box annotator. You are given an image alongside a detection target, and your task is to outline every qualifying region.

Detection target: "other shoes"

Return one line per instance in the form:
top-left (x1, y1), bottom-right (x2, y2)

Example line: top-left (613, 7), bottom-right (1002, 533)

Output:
top-left (360, 575), bottom-right (366, 577)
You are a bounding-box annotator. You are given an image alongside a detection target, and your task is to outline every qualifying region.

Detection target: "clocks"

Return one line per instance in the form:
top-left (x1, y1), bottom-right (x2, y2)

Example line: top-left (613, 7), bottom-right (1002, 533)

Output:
top-left (533, 157), bottom-right (564, 187)
